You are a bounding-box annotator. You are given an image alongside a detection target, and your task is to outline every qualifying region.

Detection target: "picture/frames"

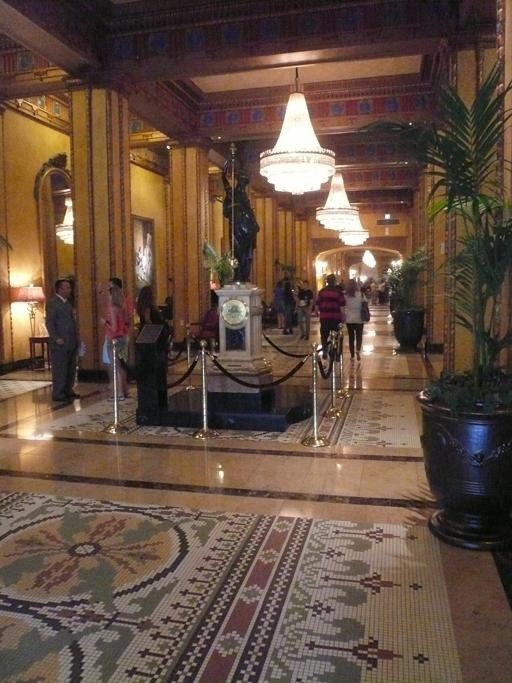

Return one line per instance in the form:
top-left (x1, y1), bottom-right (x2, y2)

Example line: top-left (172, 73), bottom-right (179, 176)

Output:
top-left (132, 214), bottom-right (157, 323)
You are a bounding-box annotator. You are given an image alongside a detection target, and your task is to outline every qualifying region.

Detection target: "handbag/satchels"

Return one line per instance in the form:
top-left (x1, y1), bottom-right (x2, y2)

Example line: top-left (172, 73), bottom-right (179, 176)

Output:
top-left (361, 292), bottom-right (370, 322)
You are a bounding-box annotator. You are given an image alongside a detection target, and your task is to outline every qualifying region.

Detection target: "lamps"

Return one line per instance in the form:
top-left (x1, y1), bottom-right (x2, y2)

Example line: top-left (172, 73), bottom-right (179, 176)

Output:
top-left (315, 171), bottom-right (359, 232)
top-left (55, 196), bottom-right (74, 246)
top-left (338, 214), bottom-right (370, 246)
top-left (14, 283), bottom-right (46, 337)
top-left (259, 66), bottom-right (336, 196)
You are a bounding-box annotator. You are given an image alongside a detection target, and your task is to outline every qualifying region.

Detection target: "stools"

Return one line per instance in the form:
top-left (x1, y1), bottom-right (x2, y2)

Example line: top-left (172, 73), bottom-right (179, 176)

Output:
top-left (29, 337), bottom-right (50, 371)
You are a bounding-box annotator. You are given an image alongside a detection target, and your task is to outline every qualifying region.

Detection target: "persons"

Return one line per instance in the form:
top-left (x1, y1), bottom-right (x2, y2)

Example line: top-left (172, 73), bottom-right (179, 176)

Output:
top-left (222, 153), bottom-right (260, 281)
top-left (107, 276), bottom-right (132, 398)
top-left (100, 283), bottom-right (128, 401)
top-left (342, 277), bottom-right (369, 361)
top-left (272, 272), bottom-right (391, 340)
top-left (315, 274), bottom-right (346, 357)
top-left (138, 284), bottom-right (164, 324)
top-left (44, 279), bottom-right (83, 400)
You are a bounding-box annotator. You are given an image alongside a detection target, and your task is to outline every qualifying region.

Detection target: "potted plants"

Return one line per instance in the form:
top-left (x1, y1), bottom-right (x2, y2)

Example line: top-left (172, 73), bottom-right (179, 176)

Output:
top-left (356, 56), bottom-right (512, 551)
top-left (378, 246), bottom-right (433, 352)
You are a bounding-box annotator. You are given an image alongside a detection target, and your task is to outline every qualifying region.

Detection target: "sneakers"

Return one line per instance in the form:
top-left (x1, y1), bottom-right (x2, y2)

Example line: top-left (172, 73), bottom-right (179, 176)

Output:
top-left (55, 393), bottom-right (130, 403)
top-left (349, 351), bottom-right (361, 361)
top-left (282, 328), bottom-right (310, 340)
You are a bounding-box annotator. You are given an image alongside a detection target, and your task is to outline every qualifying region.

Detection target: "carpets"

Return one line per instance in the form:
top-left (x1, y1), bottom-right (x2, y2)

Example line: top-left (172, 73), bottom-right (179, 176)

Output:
top-left (0, 380), bottom-right (53, 403)
top-left (0, 490), bottom-right (512, 682)
top-left (36, 302), bottom-right (432, 451)
top-left (0, 367), bottom-right (53, 381)
